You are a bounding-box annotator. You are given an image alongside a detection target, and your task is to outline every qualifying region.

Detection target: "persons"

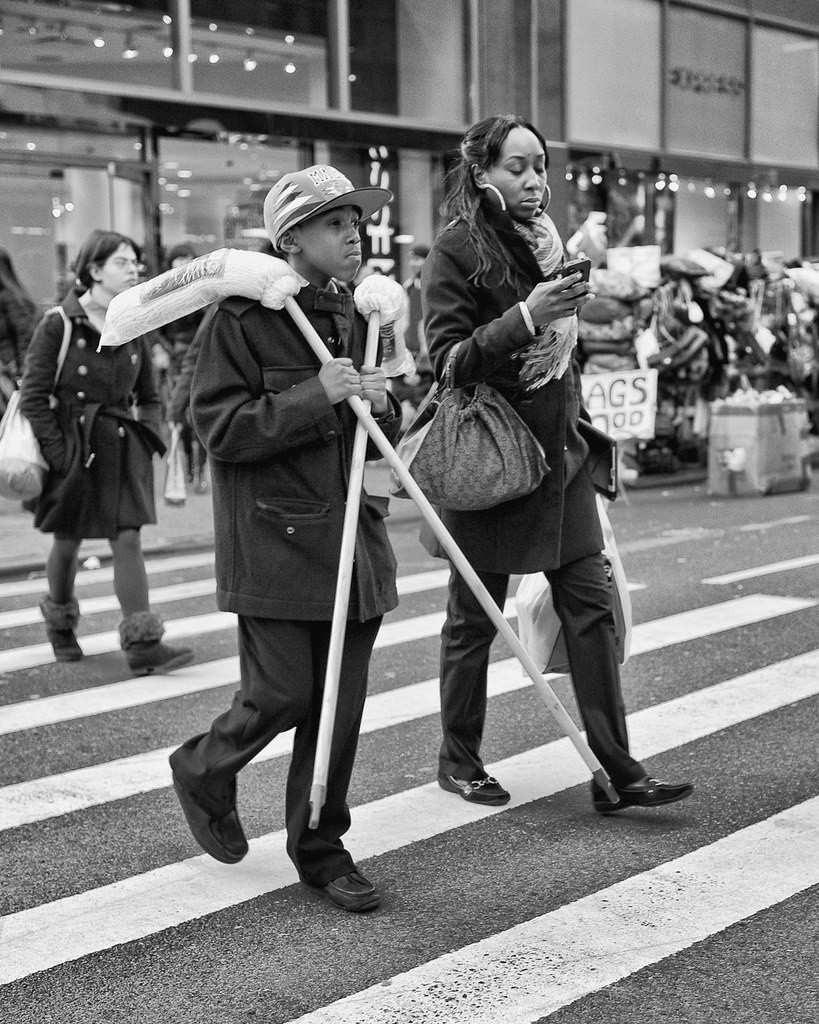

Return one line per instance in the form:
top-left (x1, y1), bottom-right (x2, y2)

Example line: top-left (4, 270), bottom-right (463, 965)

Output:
top-left (402, 244), bottom-right (430, 356)
top-left (150, 244), bottom-right (211, 494)
top-left (0, 243), bottom-right (39, 417)
top-left (420, 113), bottom-right (695, 813)
top-left (18, 227), bottom-right (195, 675)
top-left (169, 164), bottom-right (404, 913)
top-left (567, 212), bottom-right (609, 268)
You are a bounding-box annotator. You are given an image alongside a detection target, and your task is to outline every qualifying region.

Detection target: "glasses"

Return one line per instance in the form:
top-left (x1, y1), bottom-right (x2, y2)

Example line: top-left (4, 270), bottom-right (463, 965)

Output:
top-left (96, 257), bottom-right (139, 272)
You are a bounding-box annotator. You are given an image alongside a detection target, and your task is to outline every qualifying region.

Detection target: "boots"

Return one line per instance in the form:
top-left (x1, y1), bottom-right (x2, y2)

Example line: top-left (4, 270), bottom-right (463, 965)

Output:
top-left (117, 612), bottom-right (194, 676)
top-left (39, 593), bottom-right (85, 662)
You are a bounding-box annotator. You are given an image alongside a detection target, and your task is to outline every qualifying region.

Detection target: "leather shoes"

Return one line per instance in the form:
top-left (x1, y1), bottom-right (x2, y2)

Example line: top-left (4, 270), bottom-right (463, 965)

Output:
top-left (303, 870), bottom-right (382, 912)
top-left (437, 766), bottom-right (510, 804)
top-left (172, 770), bottom-right (250, 863)
top-left (592, 776), bottom-right (692, 811)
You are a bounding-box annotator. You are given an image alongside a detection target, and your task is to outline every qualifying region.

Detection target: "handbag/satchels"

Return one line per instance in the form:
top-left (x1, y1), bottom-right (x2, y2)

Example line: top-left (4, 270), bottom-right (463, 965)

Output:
top-left (389, 344), bottom-right (551, 509)
top-left (0, 391), bottom-right (55, 500)
top-left (514, 494), bottom-right (631, 674)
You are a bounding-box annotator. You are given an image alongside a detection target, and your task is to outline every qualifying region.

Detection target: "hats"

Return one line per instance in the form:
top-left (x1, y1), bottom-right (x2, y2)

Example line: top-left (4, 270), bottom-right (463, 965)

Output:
top-left (263, 165), bottom-right (393, 252)
top-left (409, 245), bottom-right (431, 258)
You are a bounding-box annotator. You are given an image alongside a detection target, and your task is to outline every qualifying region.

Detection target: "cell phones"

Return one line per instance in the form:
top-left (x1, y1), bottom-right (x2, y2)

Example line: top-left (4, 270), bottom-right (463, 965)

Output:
top-left (563, 257), bottom-right (591, 311)
